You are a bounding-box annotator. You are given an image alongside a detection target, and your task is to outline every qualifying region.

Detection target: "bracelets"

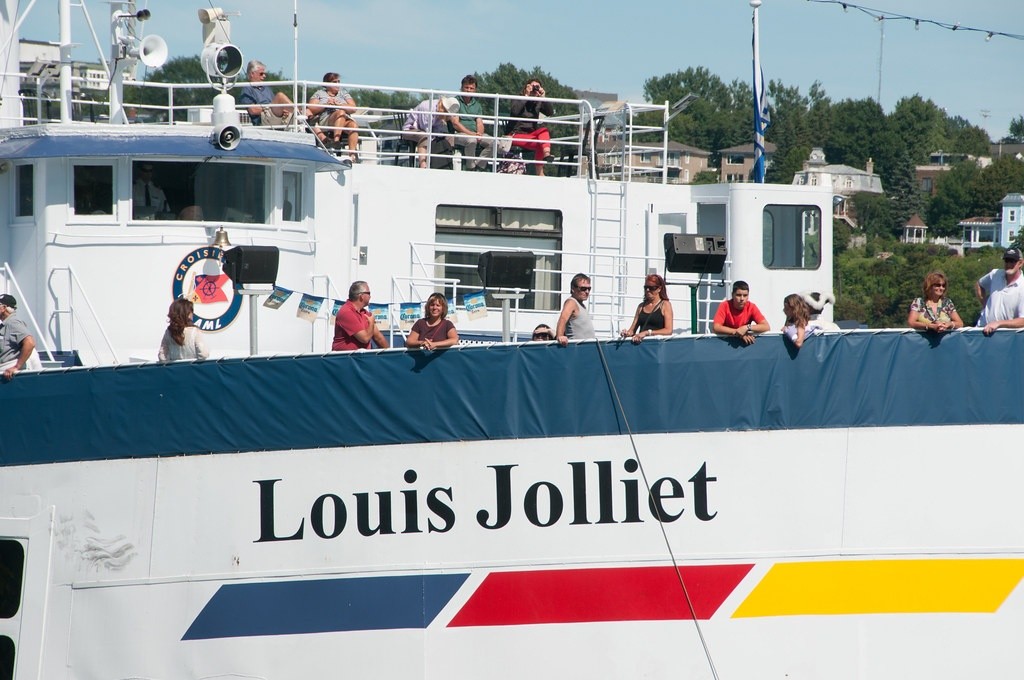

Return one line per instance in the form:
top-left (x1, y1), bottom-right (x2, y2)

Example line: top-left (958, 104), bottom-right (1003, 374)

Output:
top-left (925, 322), bottom-right (930, 330)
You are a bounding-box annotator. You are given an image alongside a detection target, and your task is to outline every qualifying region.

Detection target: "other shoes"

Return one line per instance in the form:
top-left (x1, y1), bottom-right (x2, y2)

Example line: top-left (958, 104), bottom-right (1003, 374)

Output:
top-left (334, 140), bottom-right (342, 156)
top-left (323, 137), bottom-right (332, 147)
top-left (543, 155), bottom-right (554, 162)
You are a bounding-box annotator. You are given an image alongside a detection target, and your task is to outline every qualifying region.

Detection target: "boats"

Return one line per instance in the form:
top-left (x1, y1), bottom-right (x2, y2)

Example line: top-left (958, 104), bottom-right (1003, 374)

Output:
top-left (0, 1), bottom-right (1023, 680)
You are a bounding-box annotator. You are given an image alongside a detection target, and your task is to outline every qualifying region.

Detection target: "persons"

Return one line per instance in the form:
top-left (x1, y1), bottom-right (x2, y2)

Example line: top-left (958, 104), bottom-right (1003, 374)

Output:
top-left (450, 75), bottom-right (500, 172)
top-left (975, 247), bottom-right (1024, 336)
top-left (557, 272), bottom-right (596, 346)
top-left (0, 293), bottom-right (43, 380)
top-left (402, 96), bottom-right (460, 168)
top-left (781, 291), bottom-right (811, 348)
top-left (158, 298), bottom-right (210, 362)
top-left (620, 274), bottom-right (673, 345)
top-left (907, 271), bottom-right (964, 333)
top-left (504, 78), bottom-right (555, 176)
top-left (405, 293), bottom-right (458, 351)
top-left (307, 72), bottom-right (359, 163)
top-left (532, 323), bottom-right (556, 341)
top-left (133, 161), bottom-right (171, 220)
top-left (240, 59), bottom-right (341, 150)
top-left (331, 280), bottom-right (390, 351)
top-left (713, 280), bottom-right (771, 345)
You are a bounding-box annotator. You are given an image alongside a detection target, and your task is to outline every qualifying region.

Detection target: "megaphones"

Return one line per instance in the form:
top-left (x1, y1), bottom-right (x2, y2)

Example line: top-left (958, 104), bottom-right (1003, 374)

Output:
top-left (198, 8), bottom-right (223, 23)
top-left (129, 34), bottom-right (168, 68)
top-left (118, 9), bottom-right (150, 21)
top-left (213, 125), bottom-right (241, 150)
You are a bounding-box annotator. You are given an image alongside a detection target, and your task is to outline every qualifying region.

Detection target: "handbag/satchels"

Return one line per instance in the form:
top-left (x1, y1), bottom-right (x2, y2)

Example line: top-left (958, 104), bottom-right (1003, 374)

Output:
top-left (498, 156), bottom-right (525, 174)
top-left (497, 137), bottom-right (511, 155)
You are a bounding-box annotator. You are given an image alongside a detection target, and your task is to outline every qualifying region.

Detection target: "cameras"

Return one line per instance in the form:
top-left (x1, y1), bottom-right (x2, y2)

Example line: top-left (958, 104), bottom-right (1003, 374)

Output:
top-left (533, 85), bottom-right (539, 91)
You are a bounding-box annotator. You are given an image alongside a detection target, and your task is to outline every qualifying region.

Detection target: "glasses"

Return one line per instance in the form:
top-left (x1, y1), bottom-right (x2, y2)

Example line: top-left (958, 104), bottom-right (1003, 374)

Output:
top-left (357, 292), bottom-right (370, 295)
top-left (644, 286), bottom-right (659, 290)
top-left (933, 284), bottom-right (945, 287)
top-left (1005, 259), bottom-right (1016, 263)
top-left (575, 286), bottom-right (591, 291)
top-left (257, 72), bottom-right (266, 76)
top-left (536, 333), bottom-right (547, 337)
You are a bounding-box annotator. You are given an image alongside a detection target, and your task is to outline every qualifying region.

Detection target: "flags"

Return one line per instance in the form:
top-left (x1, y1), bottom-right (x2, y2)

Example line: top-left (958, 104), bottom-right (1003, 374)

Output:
top-left (748, 0), bottom-right (771, 184)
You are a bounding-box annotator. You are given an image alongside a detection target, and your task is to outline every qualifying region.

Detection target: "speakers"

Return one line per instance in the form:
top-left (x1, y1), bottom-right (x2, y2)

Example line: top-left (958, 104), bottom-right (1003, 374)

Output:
top-left (477, 251), bottom-right (533, 288)
top-left (222, 246), bottom-right (279, 283)
top-left (664, 233), bottom-right (727, 274)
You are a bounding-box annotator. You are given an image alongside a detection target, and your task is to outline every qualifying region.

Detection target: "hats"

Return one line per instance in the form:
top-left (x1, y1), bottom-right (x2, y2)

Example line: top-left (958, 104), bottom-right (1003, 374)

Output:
top-left (1003, 247), bottom-right (1023, 261)
top-left (442, 97), bottom-right (460, 113)
top-left (0, 294), bottom-right (16, 307)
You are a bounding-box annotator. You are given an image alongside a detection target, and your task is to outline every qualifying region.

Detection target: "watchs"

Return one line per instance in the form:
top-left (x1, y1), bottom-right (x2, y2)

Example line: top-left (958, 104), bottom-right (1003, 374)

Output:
top-left (747, 324), bottom-right (752, 330)
top-left (648, 328), bottom-right (652, 336)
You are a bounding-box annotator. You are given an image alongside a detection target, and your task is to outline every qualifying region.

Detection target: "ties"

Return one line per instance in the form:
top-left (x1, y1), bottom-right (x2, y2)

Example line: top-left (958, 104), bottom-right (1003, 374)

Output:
top-left (145, 184), bottom-right (151, 206)
